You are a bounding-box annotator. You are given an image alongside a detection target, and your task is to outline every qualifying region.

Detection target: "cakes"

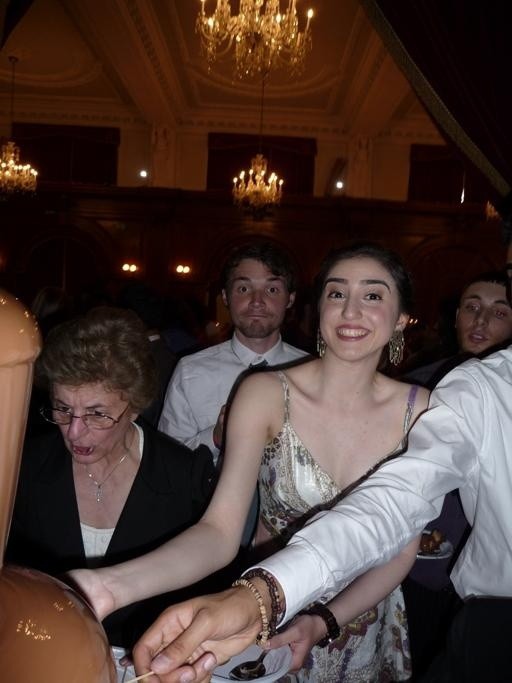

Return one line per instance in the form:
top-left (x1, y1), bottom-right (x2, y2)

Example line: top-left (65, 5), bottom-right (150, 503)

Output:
top-left (0, 291), bottom-right (119, 683)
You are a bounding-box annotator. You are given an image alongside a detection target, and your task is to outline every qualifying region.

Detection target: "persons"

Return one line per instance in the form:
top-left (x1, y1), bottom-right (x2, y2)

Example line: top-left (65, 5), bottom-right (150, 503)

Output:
top-left (157, 243), bottom-right (317, 471)
top-left (0, 306), bottom-right (249, 682)
top-left (132, 262), bottom-right (507, 681)
top-left (392, 271), bottom-right (510, 681)
top-left (51, 243), bottom-right (446, 682)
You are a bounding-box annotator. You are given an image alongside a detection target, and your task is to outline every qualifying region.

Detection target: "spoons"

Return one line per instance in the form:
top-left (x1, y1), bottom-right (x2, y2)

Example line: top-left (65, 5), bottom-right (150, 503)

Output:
top-left (229, 648), bottom-right (272, 681)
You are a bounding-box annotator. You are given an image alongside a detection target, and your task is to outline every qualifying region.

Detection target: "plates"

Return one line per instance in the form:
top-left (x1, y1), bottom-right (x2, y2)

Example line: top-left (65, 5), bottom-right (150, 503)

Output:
top-left (205, 622), bottom-right (292, 683)
top-left (417, 530), bottom-right (455, 560)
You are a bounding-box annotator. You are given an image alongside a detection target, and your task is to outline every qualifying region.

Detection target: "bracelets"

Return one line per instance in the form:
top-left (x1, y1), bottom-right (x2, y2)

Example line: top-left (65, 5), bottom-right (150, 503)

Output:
top-left (242, 567), bottom-right (283, 639)
top-left (232, 578), bottom-right (269, 645)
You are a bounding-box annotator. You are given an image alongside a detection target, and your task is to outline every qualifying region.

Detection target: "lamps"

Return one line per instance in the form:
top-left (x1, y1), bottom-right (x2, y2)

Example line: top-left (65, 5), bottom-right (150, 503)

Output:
top-left (0, 55), bottom-right (39, 198)
top-left (191, 2), bottom-right (317, 92)
top-left (228, 93), bottom-right (285, 210)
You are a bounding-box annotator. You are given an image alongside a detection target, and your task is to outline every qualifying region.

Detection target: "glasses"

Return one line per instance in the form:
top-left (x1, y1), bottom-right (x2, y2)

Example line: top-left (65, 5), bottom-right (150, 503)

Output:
top-left (39, 402), bottom-right (132, 430)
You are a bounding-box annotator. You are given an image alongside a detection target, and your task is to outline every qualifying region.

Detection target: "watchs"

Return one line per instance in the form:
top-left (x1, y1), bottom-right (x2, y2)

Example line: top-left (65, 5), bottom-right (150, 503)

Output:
top-left (306, 603), bottom-right (340, 649)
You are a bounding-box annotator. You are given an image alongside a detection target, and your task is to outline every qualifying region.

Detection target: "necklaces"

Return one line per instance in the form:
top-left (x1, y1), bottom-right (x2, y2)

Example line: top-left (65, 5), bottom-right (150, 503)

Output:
top-left (80, 427), bottom-right (136, 504)
